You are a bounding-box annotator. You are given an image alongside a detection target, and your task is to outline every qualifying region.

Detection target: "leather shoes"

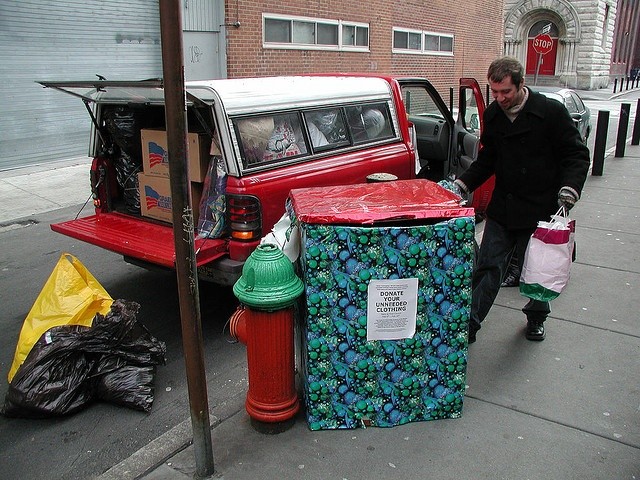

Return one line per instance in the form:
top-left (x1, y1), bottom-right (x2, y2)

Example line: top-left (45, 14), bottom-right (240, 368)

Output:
top-left (526, 318), bottom-right (546, 341)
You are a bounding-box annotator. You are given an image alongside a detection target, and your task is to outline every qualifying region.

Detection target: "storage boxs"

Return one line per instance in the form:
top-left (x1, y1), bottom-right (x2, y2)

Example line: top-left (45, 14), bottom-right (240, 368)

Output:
top-left (138, 172), bottom-right (202, 226)
top-left (141, 129), bottom-right (209, 183)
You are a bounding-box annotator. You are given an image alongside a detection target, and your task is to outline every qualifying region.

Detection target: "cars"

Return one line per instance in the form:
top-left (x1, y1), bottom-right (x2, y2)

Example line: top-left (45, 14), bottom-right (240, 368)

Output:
top-left (527, 88), bottom-right (595, 155)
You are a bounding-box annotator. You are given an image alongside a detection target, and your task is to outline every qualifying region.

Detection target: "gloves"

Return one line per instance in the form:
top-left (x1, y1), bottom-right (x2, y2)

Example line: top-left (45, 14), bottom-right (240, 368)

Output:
top-left (558, 190), bottom-right (576, 210)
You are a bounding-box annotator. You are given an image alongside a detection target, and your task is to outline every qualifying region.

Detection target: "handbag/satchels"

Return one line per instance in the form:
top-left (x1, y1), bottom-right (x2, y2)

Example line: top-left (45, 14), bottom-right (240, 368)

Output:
top-left (519, 205), bottom-right (576, 303)
top-left (550, 205), bottom-right (575, 263)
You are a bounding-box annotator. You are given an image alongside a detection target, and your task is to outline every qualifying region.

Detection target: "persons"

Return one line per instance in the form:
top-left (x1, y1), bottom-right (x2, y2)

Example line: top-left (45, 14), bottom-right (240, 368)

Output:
top-left (447, 55), bottom-right (592, 345)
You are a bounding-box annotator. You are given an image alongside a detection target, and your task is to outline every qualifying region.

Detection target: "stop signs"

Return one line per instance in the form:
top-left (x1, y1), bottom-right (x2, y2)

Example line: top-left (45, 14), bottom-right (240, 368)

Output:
top-left (532, 33), bottom-right (553, 54)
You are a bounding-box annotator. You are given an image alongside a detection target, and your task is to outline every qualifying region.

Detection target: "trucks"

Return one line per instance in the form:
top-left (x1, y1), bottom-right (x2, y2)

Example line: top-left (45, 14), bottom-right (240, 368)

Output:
top-left (35, 71), bottom-right (499, 286)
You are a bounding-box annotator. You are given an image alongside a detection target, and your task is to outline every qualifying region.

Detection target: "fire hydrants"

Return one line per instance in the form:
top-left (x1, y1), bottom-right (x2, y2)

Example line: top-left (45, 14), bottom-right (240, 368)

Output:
top-left (227, 245), bottom-right (305, 432)
